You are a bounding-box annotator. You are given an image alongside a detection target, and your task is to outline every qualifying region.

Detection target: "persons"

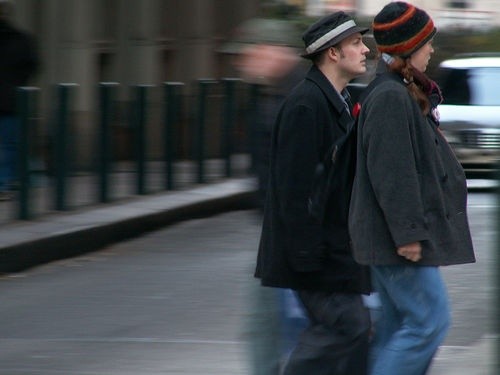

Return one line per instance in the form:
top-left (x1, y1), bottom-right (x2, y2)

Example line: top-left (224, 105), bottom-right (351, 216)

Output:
top-left (0, 2), bottom-right (40, 192)
top-left (349, 1), bottom-right (475, 375)
top-left (219, 18), bottom-right (315, 375)
top-left (251, 12), bottom-right (377, 375)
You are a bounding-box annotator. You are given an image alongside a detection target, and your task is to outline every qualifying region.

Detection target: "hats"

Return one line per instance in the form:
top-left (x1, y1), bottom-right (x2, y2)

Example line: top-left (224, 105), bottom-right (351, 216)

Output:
top-left (299, 10), bottom-right (370, 61)
top-left (371, 2), bottom-right (437, 56)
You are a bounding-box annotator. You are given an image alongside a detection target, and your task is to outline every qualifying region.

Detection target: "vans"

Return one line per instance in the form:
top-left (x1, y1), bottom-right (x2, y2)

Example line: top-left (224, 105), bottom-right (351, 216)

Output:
top-left (437, 56), bottom-right (500, 191)
